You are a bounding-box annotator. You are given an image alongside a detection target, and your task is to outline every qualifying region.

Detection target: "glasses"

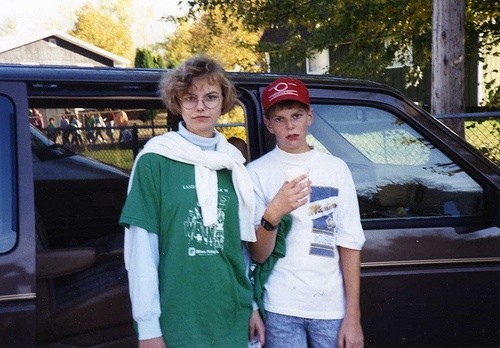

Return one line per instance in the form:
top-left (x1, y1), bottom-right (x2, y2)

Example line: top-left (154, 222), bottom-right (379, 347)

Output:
top-left (177, 93), bottom-right (223, 109)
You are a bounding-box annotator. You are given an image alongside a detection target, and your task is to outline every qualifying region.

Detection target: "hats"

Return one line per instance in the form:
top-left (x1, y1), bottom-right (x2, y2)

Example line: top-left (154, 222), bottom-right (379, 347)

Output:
top-left (261, 77), bottom-right (310, 112)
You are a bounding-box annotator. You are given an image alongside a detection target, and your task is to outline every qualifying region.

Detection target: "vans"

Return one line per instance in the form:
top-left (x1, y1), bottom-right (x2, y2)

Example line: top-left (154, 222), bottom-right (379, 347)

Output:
top-left (0, 61), bottom-right (499, 348)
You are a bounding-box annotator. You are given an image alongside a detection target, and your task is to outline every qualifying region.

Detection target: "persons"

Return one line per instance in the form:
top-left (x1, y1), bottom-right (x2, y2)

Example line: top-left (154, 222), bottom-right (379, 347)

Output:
top-left (29, 107), bottom-right (44, 130)
top-left (44, 117), bottom-right (58, 143)
top-left (59, 114), bottom-right (83, 145)
top-left (86, 112), bottom-right (95, 144)
top-left (103, 108), bottom-right (129, 144)
top-left (94, 112), bottom-right (107, 145)
top-left (247, 77), bottom-right (365, 348)
top-left (121, 55), bottom-right (266, 348)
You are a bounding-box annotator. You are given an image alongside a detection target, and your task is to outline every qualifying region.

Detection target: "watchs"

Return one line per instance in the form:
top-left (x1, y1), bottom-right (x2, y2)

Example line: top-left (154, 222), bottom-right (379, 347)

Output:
top-left (261, 216), bottom-right (281, 231)
